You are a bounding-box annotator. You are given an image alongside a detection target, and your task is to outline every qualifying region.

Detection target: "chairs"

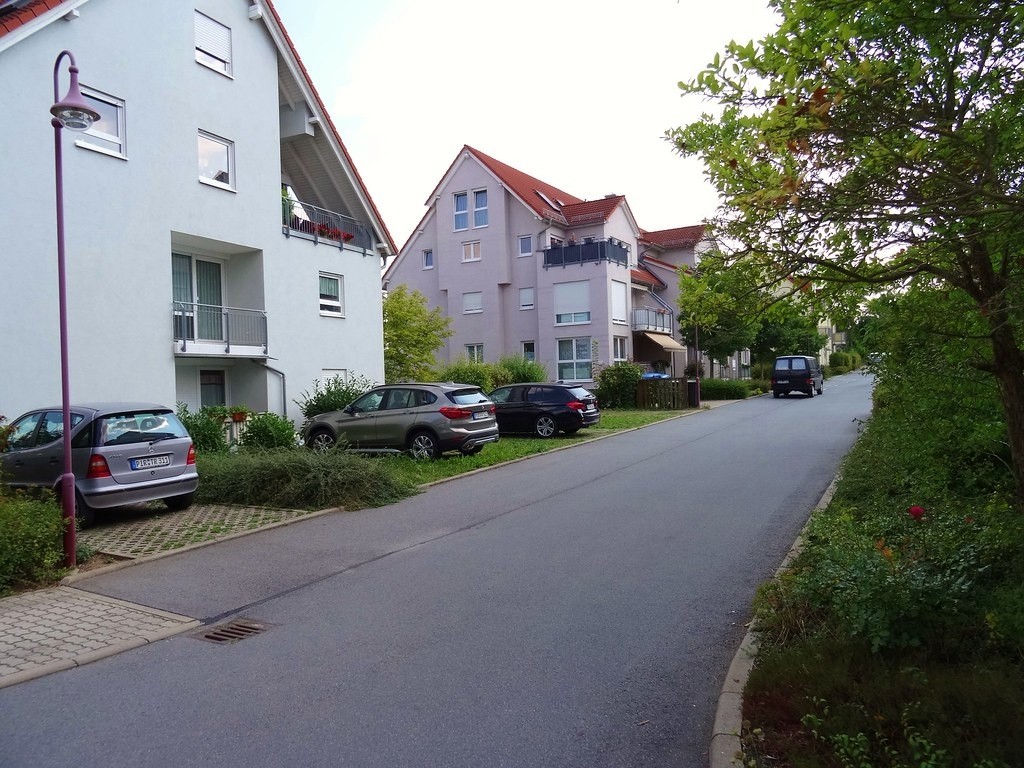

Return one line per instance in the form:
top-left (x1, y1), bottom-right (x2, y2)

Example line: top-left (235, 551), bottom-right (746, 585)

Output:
top-left (388, 391), bottom-right (406, 408)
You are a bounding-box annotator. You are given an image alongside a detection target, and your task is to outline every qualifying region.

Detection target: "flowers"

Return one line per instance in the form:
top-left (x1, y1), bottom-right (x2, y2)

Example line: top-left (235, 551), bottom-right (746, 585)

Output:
top-left (312, 224), bottom-right (354, 243)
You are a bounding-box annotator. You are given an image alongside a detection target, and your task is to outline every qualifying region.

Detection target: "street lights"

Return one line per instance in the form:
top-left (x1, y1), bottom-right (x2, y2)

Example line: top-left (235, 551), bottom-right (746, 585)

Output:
top-left (50, 50), bottom-right (100, 568)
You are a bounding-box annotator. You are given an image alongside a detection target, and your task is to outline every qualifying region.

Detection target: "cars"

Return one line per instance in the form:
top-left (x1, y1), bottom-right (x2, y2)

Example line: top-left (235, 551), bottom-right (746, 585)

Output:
top-left (304, 380), bottom-right (499, 464)
top-left (867, 353), bottom-right (882, 366)
top-left (0, 402), bottom-right (199, 531)
top-left (486, 381), bottom-right (601, 440)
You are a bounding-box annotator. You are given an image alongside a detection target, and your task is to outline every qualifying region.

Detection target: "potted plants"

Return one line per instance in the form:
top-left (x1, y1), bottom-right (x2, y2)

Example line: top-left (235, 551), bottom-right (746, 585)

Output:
top-left (228, 404), bottom-right (248, 422)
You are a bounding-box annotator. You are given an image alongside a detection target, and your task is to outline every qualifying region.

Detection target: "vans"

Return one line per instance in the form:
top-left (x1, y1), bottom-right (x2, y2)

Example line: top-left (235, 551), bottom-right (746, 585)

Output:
top-left (771, 356), bottom-right (824, 398)
